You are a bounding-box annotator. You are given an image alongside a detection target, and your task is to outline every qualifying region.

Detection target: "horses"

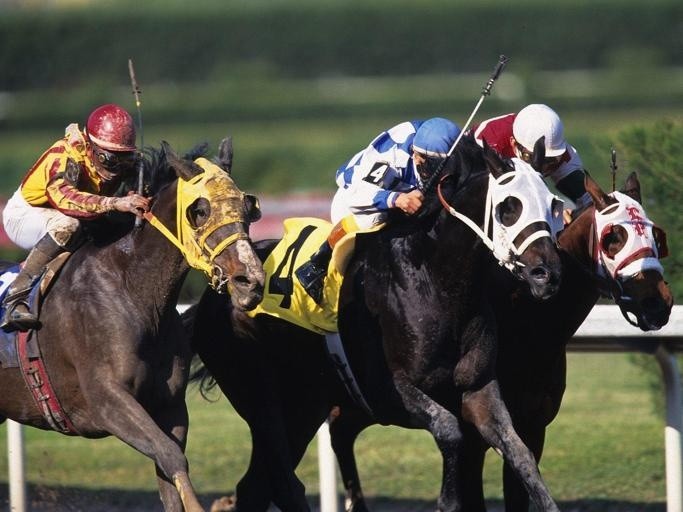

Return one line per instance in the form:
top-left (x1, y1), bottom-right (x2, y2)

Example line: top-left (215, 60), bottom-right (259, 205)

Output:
top-left (0, 135), bottom-right (267, 512)
top-left (180, 128), bottom-right (674, 512)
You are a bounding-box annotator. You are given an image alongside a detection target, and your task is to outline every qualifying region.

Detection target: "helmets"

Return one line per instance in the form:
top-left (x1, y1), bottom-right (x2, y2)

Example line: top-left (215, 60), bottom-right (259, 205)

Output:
top-left (513, 104), bottom-right (567, 158)
top-left (411, 118), bottom-right (461, 159)
top-left (83, 104), bottom-right (137, 152)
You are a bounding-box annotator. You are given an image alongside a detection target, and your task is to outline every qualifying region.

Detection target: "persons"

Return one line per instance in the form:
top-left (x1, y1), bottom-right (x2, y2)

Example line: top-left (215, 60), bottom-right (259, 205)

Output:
top-left (469, 101), bottom-right (595, 241)
top-left (293, 117), bottom-right (460, 307)
top-left (1, 102), bottom-right (153, 333)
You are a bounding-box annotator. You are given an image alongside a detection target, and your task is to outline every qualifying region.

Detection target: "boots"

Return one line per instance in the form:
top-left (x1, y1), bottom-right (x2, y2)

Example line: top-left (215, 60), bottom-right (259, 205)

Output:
top-left (0, 246), bottom-right (54, 334)
top-left (294, 239), bottom-right (335, 305)
